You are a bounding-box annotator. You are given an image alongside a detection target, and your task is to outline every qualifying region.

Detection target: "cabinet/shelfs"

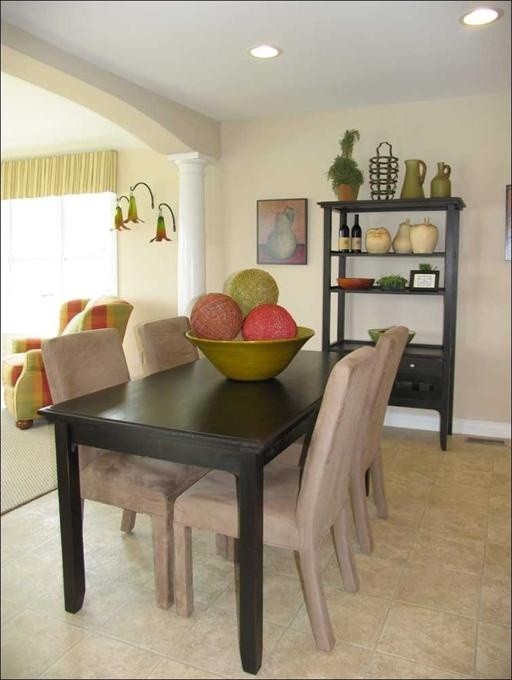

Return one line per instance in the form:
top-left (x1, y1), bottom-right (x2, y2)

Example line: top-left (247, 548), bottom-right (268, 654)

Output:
top-left (317, 197), bottom-right (467, 450)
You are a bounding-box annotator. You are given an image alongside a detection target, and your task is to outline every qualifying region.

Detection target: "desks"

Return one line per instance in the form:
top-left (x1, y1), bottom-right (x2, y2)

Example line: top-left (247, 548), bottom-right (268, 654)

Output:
top-left (35, 350), bottom-right (354, 674)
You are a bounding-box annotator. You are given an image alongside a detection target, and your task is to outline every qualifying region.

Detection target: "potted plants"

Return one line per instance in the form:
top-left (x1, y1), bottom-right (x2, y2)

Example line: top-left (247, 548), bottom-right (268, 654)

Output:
top-left (326, 129), bottom-right (364, 201)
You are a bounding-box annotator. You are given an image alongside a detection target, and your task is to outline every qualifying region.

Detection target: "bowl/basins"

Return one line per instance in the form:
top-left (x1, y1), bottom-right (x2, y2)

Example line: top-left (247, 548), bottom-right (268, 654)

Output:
top-left (336, 277), bottom-right (374, 290)
top-left (184, 328), bottom-right (315, 382)
top-left (368, 330), bottom-right (416, 347)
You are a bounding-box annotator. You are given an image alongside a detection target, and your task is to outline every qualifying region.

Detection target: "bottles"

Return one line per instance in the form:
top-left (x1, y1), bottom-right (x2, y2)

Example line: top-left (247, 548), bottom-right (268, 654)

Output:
top-left (338, 213), bottom-right (350, 253)
top-left (431, 161), bottom-right (452, 199)
top-left (351, 214), bottom-right (363, 254)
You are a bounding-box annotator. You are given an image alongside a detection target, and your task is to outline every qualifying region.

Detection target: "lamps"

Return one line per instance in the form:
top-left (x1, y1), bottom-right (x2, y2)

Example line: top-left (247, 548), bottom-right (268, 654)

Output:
top-left (149, 203), bottom-right (177, 243)
top-left (110, 196), bottom-right (131, 232)
top-left (123, 181), bottom-right (154, 224)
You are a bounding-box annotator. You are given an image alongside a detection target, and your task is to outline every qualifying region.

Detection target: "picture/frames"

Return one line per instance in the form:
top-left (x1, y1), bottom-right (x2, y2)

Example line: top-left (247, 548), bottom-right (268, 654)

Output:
top-left (410, 270), bottom-right (440, 291)
top-left (256, 198), bottom-right (308, 265)
top-left (504, 184), bottom-right (512, 262)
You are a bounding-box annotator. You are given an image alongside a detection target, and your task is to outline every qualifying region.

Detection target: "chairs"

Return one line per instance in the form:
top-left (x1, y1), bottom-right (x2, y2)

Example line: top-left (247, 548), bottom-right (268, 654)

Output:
top-left (173, 344), bottom-right (377, 653)
top-left (1, 296), bottom-right (134, 430)
top-left (276, 325), bottom-right (410, 554)
top-left (41, 327), bottom-right (212, 609)
top-left (133, 317), bottom-right (199, 376)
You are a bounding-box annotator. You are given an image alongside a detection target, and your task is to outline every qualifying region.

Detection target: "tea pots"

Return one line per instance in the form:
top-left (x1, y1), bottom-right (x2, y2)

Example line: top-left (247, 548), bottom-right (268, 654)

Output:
top-left (400, 159), bottom-right (427, 200)
